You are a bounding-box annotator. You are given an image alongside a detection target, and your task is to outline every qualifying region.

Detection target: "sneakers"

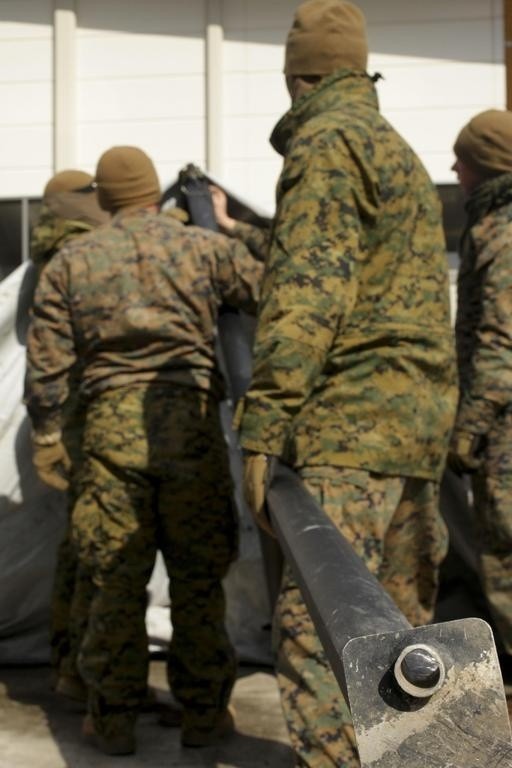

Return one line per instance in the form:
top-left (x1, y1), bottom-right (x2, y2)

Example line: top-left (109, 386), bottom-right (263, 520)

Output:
top-left (79, 713), bottom-right (136, 756)
top-left (180, 701), bottom-right (234, 748)
top-left (47, 667), bottom-right (171, 716)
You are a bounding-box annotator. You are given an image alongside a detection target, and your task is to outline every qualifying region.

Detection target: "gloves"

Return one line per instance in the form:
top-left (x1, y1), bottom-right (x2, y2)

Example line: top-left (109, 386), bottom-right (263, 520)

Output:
top-left (243, 454), bottom-right (279, 539)
top-left (30, 440), bottom-right (75, 493)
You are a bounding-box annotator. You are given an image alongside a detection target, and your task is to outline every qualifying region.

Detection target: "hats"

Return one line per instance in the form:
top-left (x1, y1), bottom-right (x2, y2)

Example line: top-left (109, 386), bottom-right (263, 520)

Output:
top-left (96, 146), bottom-right (162, 211)
top-left (453, 109), bottom-right (512, 177)
top-left (284, 0), bottom-right (369, 79)
top-left (41, 170), bottom-right (111, 229)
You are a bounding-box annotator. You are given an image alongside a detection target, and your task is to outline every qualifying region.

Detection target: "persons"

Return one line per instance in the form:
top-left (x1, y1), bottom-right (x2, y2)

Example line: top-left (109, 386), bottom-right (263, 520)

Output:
top-left (1, 148), bottom-right (275, 758)
top-left (439, 109), bottom-right (511, 696)
top-left (231, 1), bottom-right (463, 768)
